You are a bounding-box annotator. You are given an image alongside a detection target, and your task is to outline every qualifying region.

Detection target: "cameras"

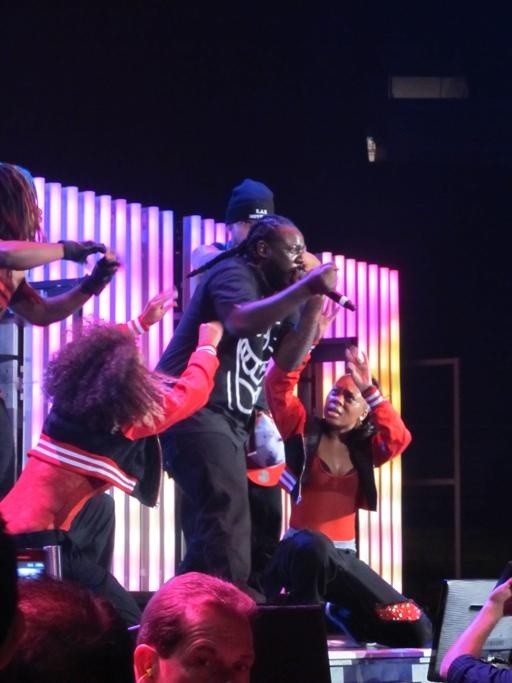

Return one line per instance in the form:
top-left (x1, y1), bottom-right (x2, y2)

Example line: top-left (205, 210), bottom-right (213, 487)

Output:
top-left (13, 544), bottom-right (62, 582)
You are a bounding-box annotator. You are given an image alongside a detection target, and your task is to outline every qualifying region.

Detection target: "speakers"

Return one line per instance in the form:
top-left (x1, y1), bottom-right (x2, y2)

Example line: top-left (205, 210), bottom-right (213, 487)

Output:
top-left (127, 606), bottom-right (331, 683)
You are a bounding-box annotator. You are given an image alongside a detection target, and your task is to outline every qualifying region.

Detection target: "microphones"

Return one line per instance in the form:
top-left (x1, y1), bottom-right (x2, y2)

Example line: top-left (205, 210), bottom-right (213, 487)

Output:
top-left (298, 270), bottom-right (356, 312)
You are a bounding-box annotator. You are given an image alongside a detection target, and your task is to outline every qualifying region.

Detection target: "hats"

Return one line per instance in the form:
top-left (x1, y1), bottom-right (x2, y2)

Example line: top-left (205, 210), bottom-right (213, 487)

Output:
top-left (224, 178), bottom-right (276, 224)
top-left (246, 412), bottom-right (287, 487)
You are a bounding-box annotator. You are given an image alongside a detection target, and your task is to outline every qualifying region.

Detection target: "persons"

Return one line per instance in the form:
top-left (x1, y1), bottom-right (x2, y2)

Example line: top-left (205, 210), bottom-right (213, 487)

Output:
top-left (148, 211), bottom-right (339, 608)
top-left (437, 575), bottom-right (511, 682)
top-left (133, 570), bottom-right (261, 682)
top-left (1, 158), bottom-right (125, 484)
top-left (264, 293), bottom-right (438, 649)
top-left (0, 574), bottom-right (136, 683)
top-left (189, 175), bottom-right (324, 590)
top-left (0, 286), bottom-right (224, 632)
